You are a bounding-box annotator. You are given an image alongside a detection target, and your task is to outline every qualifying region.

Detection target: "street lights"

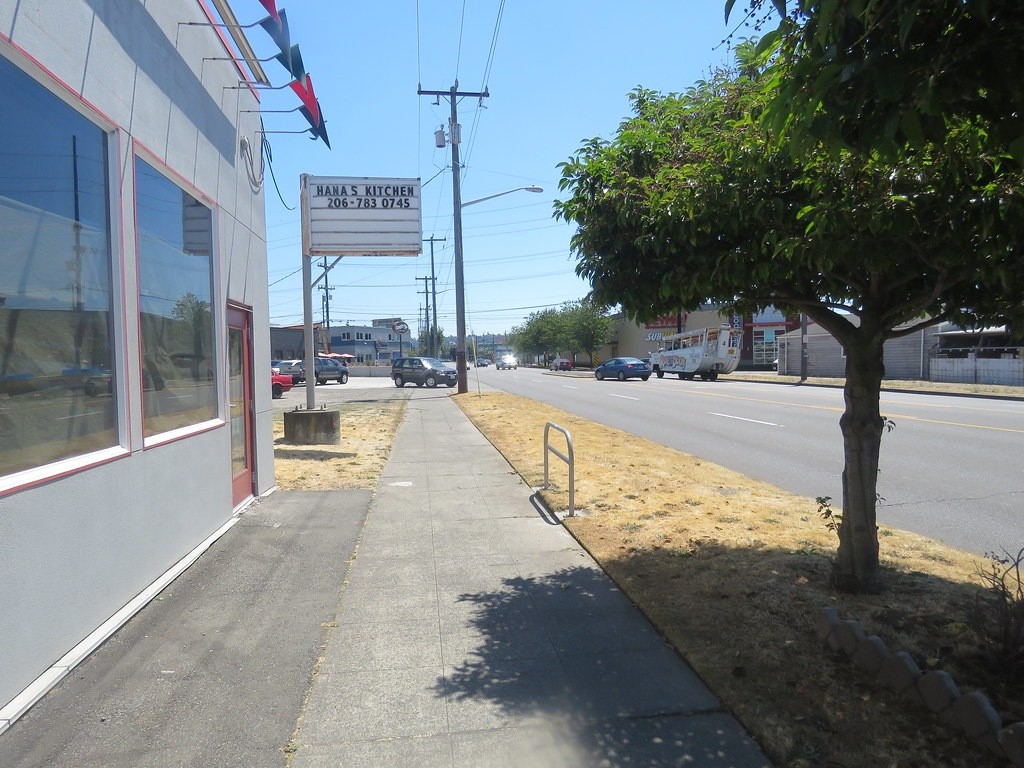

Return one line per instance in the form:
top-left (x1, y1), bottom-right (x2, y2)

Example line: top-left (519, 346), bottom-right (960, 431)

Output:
top-left (454, 186), bottom-right (545, 388)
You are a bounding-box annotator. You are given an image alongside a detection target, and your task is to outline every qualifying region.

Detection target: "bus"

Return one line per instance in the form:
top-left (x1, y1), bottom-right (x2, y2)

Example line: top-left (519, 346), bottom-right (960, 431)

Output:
top-left (648, 323), bottom-right (743, 382)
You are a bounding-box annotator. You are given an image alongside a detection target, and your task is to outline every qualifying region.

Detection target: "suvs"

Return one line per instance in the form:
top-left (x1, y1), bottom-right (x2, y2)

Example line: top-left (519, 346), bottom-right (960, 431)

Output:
top-left (301, 358), bottom-right (350, 385)
top-left (391, 356), bottom-right (458, 388)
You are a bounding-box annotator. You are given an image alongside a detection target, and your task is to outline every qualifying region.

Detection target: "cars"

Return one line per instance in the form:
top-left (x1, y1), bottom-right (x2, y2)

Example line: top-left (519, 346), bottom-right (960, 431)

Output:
top-left (455, 360), bottom-right (470, 370)
top-left (595, 357), bottom-right (652, 381)
top-left (485, 359), bottom-right (492, 365)
top-left (496, 356), bottom-right (518, 370)
top-left (550, 358), bottom-right (572, 372)
top-left (474, 358), bottom-right (489, 368)
top-left (271, 368), bottom-right (294, 399)
top-left (271, 359), bottom-right (304, 385)
